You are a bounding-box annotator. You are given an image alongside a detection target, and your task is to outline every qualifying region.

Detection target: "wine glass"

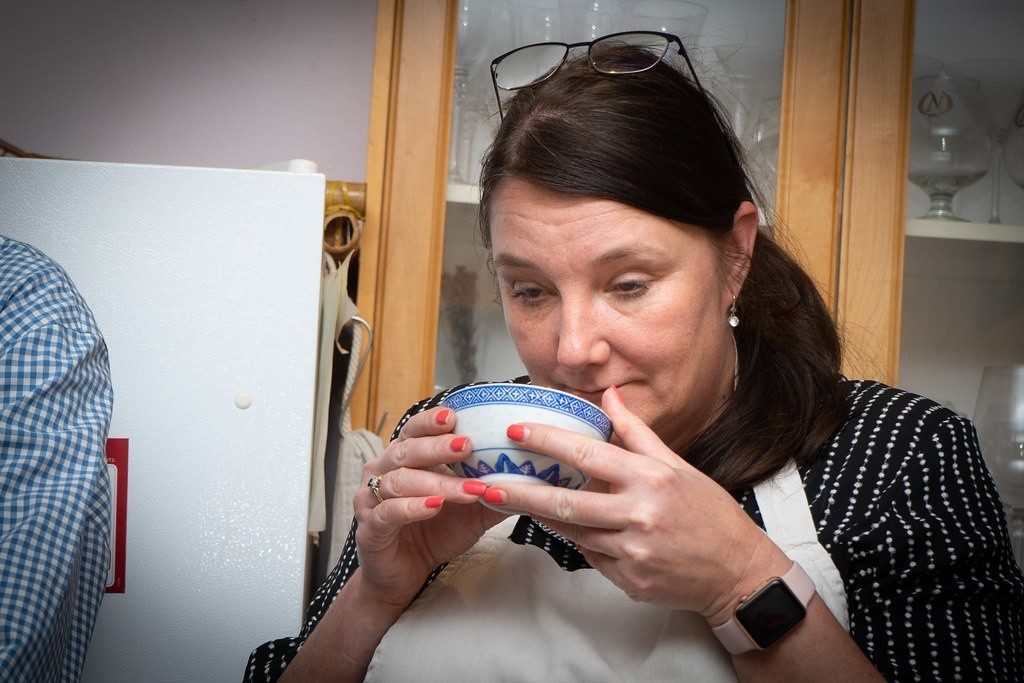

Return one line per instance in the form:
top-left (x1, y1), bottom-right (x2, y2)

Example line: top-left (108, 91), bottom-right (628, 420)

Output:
top-left (909, 54), bottom-right (1024, 223)
top-left (972, 365), bottom-right (1024, 565)
top-left (449, 0), bottom-right (784, 217)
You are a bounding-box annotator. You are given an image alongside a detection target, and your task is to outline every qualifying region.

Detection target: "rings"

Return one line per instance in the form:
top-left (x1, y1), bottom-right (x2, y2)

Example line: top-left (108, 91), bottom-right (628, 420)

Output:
top-left (367, 472), bottom-right (385, 503)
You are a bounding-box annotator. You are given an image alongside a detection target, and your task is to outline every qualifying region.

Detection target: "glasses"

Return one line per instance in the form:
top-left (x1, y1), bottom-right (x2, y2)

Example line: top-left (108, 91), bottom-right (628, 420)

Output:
top-left (490, 29), bottom-right (704, 122)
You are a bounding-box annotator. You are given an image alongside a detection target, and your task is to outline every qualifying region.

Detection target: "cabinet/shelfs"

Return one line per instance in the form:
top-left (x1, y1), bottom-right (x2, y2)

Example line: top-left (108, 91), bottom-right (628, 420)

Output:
top-left (351, 0), bottom-right (1023, 575)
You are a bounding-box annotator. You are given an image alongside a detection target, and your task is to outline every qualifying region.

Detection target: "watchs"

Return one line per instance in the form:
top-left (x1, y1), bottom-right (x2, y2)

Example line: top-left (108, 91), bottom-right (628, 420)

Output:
top-left (710, 559), bottom-right (816, 655)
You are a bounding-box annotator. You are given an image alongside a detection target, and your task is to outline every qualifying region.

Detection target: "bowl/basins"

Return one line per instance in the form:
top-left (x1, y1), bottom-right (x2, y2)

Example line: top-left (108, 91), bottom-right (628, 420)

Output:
top-left (440, 384), bottom-right (613, 515)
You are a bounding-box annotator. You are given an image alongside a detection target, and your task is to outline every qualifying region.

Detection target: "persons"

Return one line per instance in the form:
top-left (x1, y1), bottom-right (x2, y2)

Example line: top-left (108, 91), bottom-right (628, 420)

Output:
top-left (244, 31), bottom-right (1024, 683)
top-left (0, 233), bottom-right (112, 683)
